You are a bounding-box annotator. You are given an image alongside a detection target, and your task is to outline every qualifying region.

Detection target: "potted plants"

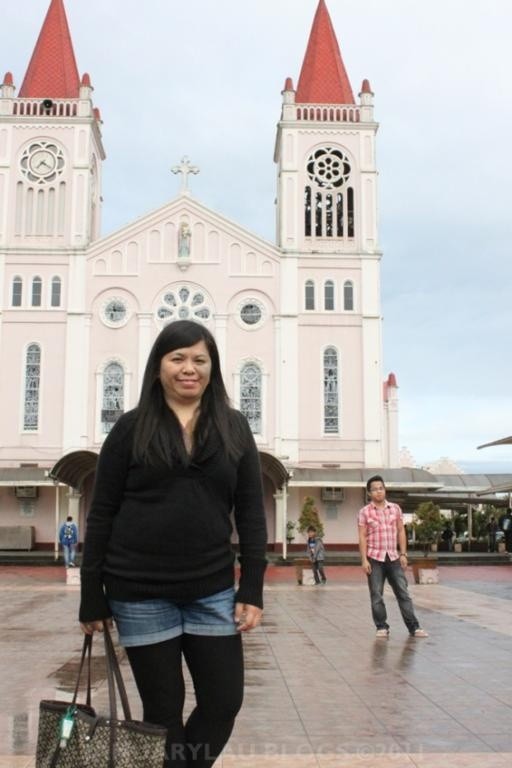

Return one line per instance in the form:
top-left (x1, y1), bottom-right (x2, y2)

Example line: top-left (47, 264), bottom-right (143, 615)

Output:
top-left (295, 497), bottom-right (324, 585)
top-left (403, 501), bottom-right (444, 584)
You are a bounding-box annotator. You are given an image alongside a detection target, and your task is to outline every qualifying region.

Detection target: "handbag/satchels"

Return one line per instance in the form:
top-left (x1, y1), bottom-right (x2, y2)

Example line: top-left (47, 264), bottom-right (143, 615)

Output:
top-left (35, 700), bottom-right (168, 768)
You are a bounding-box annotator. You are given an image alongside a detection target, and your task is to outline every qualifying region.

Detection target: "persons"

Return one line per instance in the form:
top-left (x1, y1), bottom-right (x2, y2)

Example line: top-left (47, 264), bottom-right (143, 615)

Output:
top-left (306, 524), bottom-right (327, 585)
top-left (358, 477), bottom-right (427, 639)
top-left (59, 516), bottom-right (79, 569)
top-left (77, 319), bottom-right (269, 768)
top-left (486, 508), bottom-right (512, 553)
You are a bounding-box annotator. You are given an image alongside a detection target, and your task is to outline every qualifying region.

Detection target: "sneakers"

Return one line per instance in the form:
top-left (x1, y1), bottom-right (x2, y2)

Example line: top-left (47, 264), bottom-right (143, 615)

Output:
top-left (65, 562), bottom-right (75, 569)
top-left (375, 628), bottom-right (391, 637)
top-left (413, 628), bottom-right (428, 637)
top-left (314, 580), bottom-right (327, 586)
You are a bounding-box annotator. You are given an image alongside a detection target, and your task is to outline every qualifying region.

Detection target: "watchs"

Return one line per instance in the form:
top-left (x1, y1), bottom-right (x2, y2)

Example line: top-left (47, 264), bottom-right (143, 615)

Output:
top-left (400, 553), bottom-right (408, 556)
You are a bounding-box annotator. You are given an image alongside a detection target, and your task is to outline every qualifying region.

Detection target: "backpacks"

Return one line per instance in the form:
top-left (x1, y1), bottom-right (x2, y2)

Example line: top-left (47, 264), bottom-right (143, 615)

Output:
top-left (64, 527), bottom-right (73, 540)
